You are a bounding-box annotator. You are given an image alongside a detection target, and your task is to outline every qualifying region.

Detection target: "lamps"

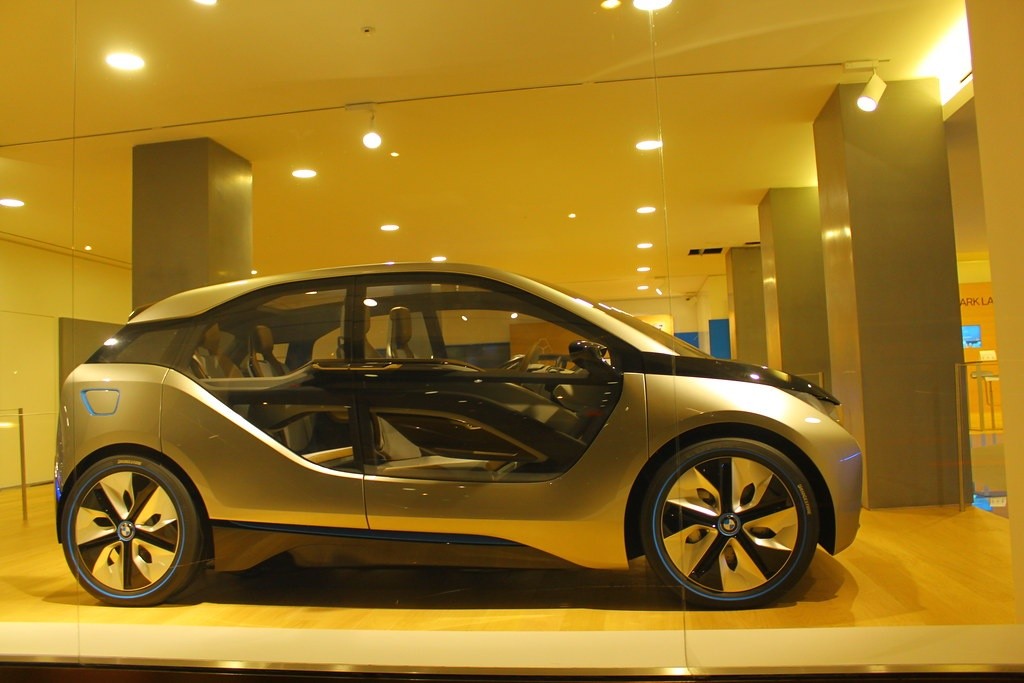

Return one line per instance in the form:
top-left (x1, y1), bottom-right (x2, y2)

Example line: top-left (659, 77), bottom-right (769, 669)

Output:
top-left (857, 73), bottom-right (887, 112)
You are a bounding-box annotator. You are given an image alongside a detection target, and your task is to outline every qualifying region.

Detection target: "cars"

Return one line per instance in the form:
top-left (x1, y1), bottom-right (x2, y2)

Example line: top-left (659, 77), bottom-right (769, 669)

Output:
top-left (52, 261), bottom-right (865, 611)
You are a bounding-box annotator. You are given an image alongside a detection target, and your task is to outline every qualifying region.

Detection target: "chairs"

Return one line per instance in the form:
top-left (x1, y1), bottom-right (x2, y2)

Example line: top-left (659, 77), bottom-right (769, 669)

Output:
top-left (387, 308), bottom-right (415, 359)
top-left (241, 325), bottom-right (322, 453)
top-left (191, 323), bottom-right (353, 464)
top-left (341, 305), bottom-right (518, 481)
